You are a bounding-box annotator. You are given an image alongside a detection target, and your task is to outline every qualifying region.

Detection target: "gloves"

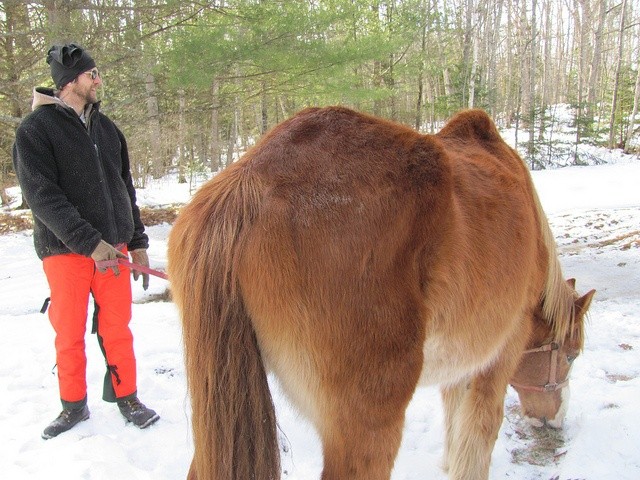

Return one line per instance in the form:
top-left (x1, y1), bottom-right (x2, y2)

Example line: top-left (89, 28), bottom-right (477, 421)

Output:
top-left (130, 249), bottom-right (150, 290)
top-left (91, 240), bottom-right (130, 276)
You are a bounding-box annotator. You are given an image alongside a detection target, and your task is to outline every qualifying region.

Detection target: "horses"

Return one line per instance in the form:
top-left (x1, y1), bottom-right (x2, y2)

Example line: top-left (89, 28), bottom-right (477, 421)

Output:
top-left (167, 109), bottom-right (596, 480)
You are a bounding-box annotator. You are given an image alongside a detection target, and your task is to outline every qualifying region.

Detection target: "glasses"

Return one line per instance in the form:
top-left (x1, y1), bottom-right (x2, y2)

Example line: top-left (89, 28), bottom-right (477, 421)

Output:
top-left (84, 69), bottom-right (100, 79)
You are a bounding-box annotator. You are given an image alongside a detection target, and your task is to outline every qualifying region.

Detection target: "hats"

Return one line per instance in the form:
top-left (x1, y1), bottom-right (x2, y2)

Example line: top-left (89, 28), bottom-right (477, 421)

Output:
top-left (46, 42), bottom-right (97, 90)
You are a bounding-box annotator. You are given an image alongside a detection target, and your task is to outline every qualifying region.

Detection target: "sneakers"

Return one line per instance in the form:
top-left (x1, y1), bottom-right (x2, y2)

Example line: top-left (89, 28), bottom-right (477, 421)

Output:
top-left (118, 397), bottom-right (160, 429)
top-left (41, 405), bottom-right (90, 440)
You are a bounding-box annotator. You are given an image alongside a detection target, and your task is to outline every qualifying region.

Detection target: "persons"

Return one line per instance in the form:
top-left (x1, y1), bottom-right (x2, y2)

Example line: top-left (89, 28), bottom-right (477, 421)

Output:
top-left (13, 43), bottom-right (161, 440)
